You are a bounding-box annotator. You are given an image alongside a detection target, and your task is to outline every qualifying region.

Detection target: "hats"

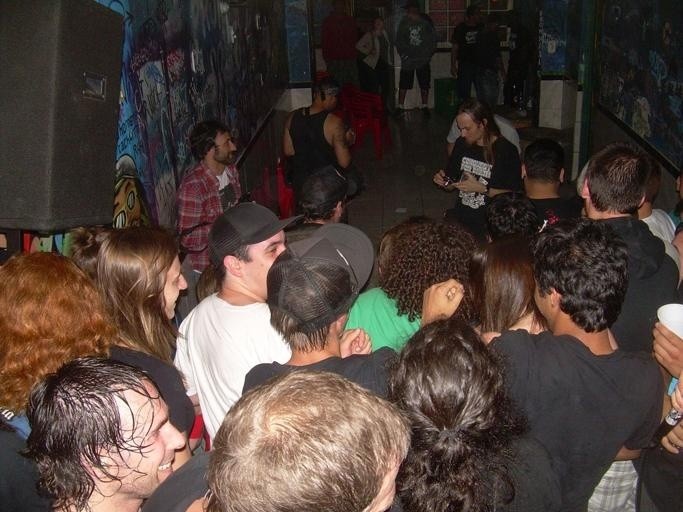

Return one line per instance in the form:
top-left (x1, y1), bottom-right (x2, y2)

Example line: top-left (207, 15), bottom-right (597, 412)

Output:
top-left (205, 200), bottom-right (308, 270)
top-left (400, 0), bottom-right (421, 9)
top-left (264, 221), bottom-right (376, 336)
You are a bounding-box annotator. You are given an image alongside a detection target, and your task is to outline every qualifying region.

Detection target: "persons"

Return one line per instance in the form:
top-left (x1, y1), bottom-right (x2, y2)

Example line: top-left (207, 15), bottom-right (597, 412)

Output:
top-left (578, 142), bottom-right (679, 356)
top-left (518, 137), bottom-right (584, 217)
top-left (175, 118), bottom-right (242, 266)
top-left (281, 78), bottom-right (361, 202)
top-left (0, 160), bottom-right (682, 512)
top-left (316, 1), bottom-right (538, 121)
top-left (446, 113), bottom-right (522, 159)
top-left (432, 98), bottom-right (518, 230)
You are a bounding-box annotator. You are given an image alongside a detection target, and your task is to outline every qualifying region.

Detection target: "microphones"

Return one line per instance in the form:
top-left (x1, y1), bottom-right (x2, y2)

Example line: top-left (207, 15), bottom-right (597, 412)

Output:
top-left (650, 406), bottom-right (682, 450)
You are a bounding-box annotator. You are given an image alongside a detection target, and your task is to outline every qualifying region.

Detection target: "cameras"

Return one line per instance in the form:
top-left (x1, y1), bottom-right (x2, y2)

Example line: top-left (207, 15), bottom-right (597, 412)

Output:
top-left (442, 176), bottom-right (455, 186)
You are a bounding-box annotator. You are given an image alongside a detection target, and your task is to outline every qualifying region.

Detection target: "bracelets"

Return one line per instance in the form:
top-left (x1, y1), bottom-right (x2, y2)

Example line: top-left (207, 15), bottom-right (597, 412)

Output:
top-left (480, 185), bottom-right (489, 195)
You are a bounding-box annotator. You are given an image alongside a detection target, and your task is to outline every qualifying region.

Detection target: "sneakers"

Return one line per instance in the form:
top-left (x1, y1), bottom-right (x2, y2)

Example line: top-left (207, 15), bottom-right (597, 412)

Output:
top-left (391, 107), bottom-right (405, 116)
top-left (422, 105), bottom-right (431, 118)
top-left (525, 98), bottom-right (536, 111)
top-left (513, 108), bottom-right (529, 119)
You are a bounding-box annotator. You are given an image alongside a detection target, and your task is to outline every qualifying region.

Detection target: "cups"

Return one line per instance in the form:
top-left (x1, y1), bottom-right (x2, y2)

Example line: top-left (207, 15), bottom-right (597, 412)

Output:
top-left (656, 302), bottom-right (682, 341)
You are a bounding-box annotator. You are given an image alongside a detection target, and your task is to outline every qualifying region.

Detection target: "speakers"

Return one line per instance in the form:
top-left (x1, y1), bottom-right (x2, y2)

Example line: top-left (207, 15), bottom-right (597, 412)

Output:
top-left (0, 0), bottom-right (124, 233)
